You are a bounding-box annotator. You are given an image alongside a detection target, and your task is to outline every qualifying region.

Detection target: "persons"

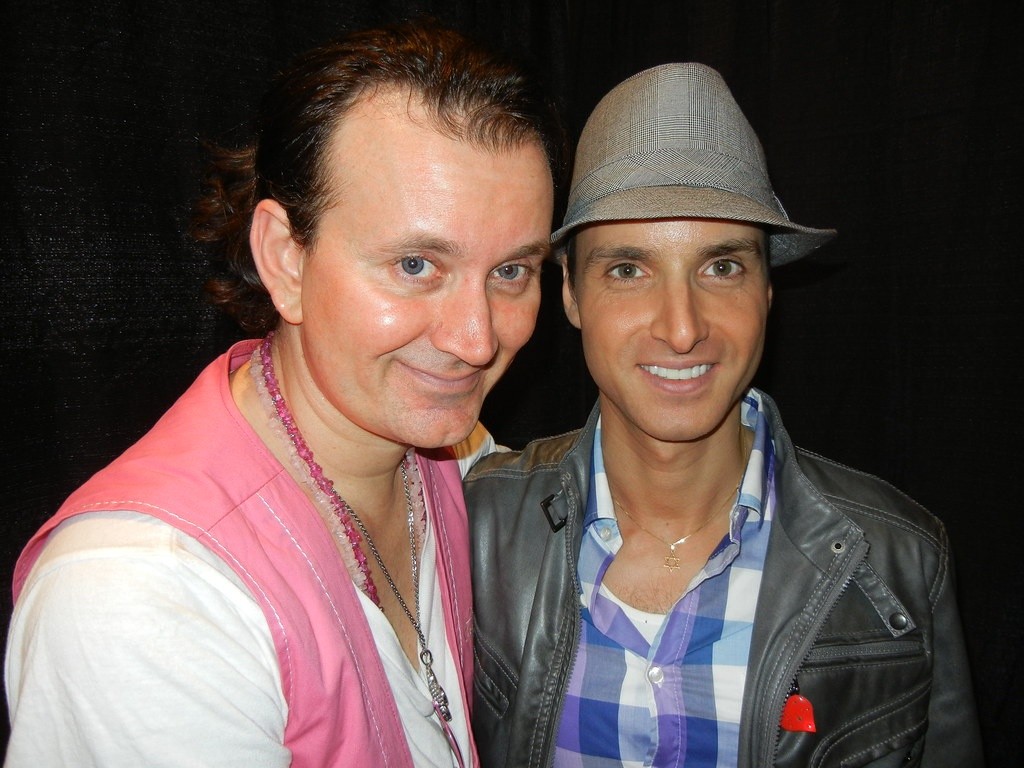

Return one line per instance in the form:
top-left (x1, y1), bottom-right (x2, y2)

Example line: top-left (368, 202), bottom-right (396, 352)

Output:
top-left (0, 17), bottom-right (563, 768)
top-left (457, 62), bottom-right (983, 768)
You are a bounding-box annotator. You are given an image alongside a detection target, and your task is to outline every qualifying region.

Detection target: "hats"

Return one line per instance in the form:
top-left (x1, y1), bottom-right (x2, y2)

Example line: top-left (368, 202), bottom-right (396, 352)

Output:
top-left (545, 62), bottom-right (837, 269)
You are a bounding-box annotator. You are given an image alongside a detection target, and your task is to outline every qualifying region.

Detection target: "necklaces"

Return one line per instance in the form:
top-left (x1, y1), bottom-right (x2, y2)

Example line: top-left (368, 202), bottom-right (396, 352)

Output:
top-left (611, 470), bottom-right (741, 574)
top-left (251, 328), bottom-right (428, 609)
top-left (334, 455), bottom-right (454, 721)
